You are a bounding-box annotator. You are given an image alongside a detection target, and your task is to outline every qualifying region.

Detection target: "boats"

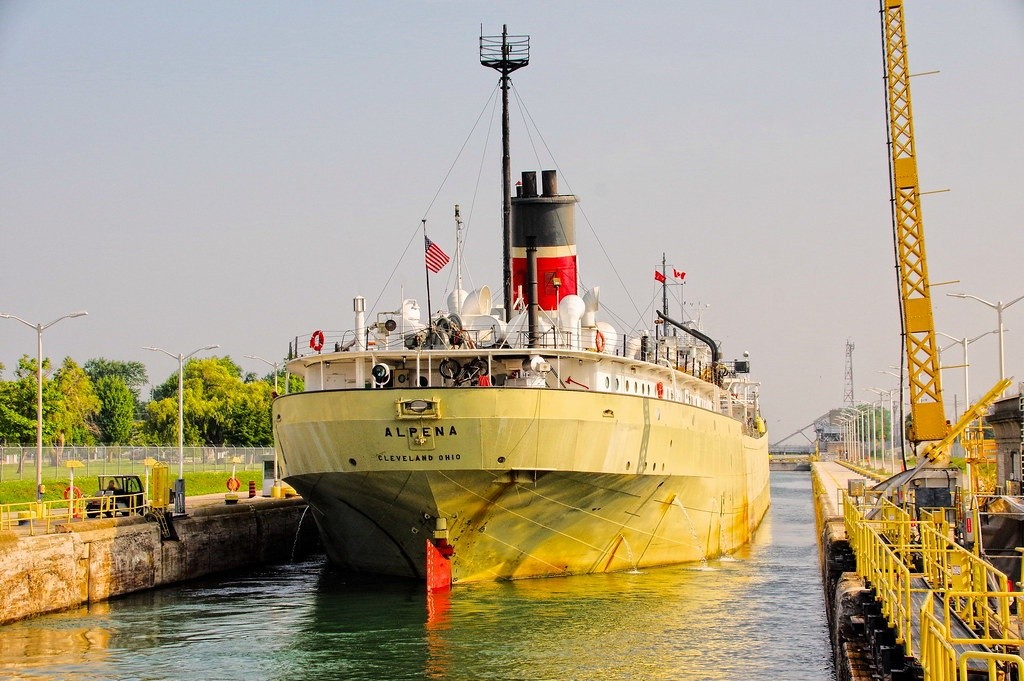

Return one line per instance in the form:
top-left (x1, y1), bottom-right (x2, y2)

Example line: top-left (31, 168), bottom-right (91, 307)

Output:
top-left (269, 19), bottom-right (769, 595)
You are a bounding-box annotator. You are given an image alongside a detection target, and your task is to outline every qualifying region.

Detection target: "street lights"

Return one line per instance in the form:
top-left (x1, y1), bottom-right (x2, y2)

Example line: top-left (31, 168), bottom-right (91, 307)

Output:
top-left (947, 292), bottom-right (1024, 402)
top-left (243, 354), bottom-right (292, 485)
top-left (934, 328), bottom-right (1011, 499)
top-left (0, 312), bottom-right (89, 502)
top-left (831, 365), bottom-right (924, 479)
top-left (140, 343), bottom-right (219, 480)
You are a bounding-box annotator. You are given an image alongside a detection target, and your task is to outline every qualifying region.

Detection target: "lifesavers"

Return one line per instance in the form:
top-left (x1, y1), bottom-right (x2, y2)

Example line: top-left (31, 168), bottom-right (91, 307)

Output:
top-left (656, 381), bottom-right (665, 399)
top-left (310, 329), bottom-right (325, 351)
top-left (439, 357), bottom-right (462, 379)
top-left (470, 355), bottom-right (491, 377)
top-left (594, 330), bottom-right (606, 351)
top-left (227, 477), bottom-right (240, 492)
top-left (63, 486), bottom-right (81, 501)
top-left (385, 318), bottom-right (397, 332)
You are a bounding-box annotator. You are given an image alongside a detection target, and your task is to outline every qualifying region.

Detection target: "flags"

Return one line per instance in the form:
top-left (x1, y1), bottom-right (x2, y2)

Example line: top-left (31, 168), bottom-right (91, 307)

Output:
top-left (655, 270), bottom-right (666, 283)
top-left (674, 268), bottom-right (686, 279)
top-left (424, 234), bottom-right (450, 273)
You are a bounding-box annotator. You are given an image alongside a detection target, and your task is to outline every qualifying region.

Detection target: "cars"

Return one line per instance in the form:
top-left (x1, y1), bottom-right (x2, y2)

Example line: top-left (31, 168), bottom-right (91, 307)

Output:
top-left (86, 474), bottom-right (145, 518)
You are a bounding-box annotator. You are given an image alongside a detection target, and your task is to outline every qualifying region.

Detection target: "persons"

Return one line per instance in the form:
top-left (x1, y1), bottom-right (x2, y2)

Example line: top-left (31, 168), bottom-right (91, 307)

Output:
top-left (107, 480), bottom-right (117, 490)
